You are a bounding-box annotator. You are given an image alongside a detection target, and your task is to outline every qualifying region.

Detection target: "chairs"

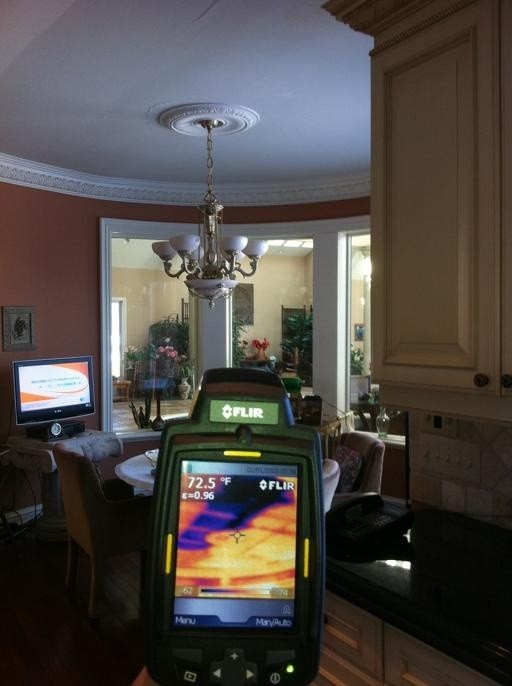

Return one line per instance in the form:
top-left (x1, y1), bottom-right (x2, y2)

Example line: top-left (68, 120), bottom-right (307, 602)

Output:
top-left (322, 430), bottom-right (387, 515)
top-left (52, 440), bottom-right (151, 623)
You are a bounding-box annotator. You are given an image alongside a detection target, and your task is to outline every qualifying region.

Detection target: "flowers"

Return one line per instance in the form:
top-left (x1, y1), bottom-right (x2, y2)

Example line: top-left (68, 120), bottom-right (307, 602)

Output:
top-left (154, 344), bottom-right (180, 364)
top-left (253, 337), bottom-right (269, 352)
top-left (174, 354), bottom-right (194, 380)
top-left (124, 343), bottom-right (140, 361)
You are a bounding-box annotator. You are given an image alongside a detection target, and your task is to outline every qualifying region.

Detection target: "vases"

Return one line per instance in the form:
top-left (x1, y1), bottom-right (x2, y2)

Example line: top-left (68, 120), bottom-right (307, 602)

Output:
top-left (177, 377), bottom-right (193, 400)
top-left (127, 360), bottom-right (135, 370)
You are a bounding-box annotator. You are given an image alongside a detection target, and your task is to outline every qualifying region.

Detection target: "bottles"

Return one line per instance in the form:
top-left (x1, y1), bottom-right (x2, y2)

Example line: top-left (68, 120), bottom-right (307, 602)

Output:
top-left (375, 407), bottom-right (390, 439)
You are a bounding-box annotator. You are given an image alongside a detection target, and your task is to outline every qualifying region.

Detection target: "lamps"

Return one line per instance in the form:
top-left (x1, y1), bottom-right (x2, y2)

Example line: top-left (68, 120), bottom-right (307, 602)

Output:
top-left (146, 100), bottom-right (270, 311)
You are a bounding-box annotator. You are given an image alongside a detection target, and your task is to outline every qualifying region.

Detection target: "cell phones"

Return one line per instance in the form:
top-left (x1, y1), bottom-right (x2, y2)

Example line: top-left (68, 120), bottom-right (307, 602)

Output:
top-left (139, 367), bottom-right (331, 686)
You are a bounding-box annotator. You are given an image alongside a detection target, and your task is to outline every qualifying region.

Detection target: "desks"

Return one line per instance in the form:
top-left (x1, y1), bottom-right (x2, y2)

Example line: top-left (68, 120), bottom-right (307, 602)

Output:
top-left (5, 429), bottom-right (125, 537)
top-left (115, 447), bottom-right (159, 494)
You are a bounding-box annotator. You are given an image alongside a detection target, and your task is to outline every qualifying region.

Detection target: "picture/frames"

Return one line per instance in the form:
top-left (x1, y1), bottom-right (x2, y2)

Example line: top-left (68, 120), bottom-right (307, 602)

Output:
top-left (1, 303), bottom-right (36, 353)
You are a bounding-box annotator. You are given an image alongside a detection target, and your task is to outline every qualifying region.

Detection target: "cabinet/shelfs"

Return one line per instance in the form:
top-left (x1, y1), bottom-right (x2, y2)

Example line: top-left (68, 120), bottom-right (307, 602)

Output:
top-left (305, 600), bottom-right (493, 686)
top-left (368, 2), bottom-right (511, 416)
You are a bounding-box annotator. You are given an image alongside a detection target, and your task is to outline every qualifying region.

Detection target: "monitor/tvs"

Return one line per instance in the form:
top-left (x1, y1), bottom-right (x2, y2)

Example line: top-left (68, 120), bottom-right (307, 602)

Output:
top-left (10, 355), bottom-right (96, 428)
